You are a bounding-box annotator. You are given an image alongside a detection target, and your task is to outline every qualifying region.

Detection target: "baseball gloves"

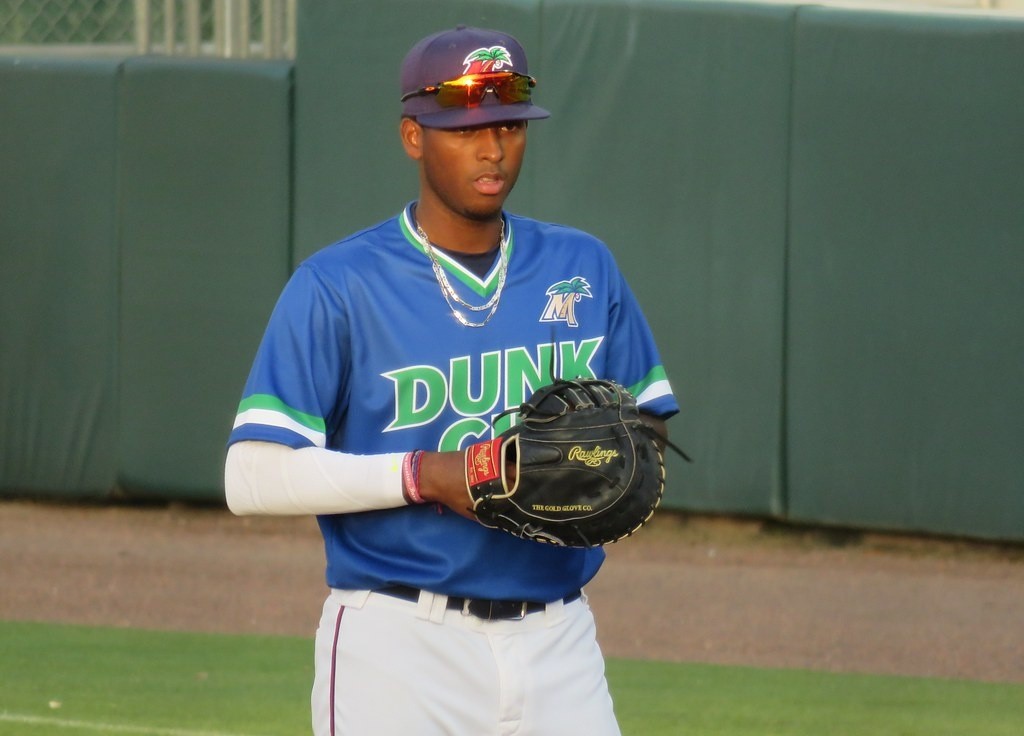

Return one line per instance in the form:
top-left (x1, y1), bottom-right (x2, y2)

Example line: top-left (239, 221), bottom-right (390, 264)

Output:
top-left (462, 377), bottom-right (696, 550)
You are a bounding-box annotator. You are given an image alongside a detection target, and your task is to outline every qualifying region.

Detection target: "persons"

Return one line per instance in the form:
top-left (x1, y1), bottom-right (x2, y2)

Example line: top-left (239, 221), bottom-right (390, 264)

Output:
top-left (225, 26), bottom-right (680, 736)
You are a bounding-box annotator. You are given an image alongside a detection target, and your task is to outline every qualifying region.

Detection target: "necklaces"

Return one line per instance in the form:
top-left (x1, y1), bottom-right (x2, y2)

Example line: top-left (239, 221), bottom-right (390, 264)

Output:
top-left (415, 219), bottom-right (508, 327)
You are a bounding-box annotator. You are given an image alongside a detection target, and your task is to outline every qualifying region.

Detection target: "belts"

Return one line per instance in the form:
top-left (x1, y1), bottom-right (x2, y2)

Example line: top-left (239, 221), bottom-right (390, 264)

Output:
top-left (369, 584), bottom-right (581, 623)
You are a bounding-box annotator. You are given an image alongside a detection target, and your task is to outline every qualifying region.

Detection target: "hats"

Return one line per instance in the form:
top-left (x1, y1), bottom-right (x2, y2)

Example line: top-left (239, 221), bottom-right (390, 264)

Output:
top-left (399, 24), bottom-right (551, 129)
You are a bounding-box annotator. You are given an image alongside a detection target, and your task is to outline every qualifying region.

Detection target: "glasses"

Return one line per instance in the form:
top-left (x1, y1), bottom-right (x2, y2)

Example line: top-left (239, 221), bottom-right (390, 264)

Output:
top-left (400, 71), bottom-right (537, 108)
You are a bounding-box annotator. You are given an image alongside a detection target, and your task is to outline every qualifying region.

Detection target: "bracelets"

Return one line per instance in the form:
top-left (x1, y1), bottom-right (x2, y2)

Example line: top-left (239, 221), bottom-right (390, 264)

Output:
top-left (402, 450), bottom-right (425, 504)
